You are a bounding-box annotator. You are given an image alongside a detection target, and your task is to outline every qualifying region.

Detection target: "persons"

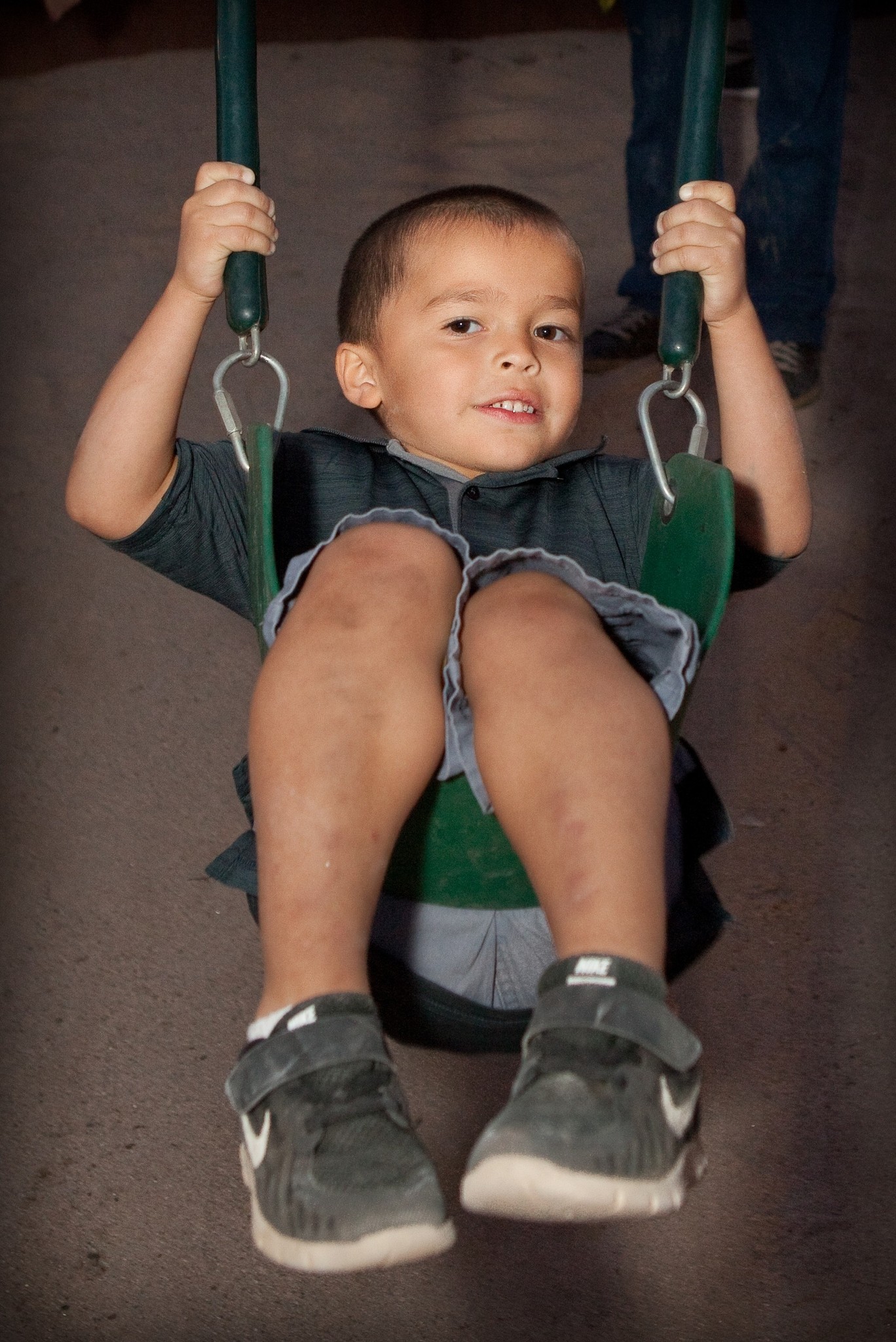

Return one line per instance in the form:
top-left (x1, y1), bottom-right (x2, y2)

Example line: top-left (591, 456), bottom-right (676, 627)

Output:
top-left (67, 164), bottom-right (813, 1275)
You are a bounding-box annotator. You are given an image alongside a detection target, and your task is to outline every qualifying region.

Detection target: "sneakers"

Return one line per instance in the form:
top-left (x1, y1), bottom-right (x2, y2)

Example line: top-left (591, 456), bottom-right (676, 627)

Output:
top-left (222, 991), bottom-right (459, 1277)
top-left (765, 333), bottom-right (831, 410)
top-left (581, 291), bottom-right (669, 368)
top-left (461, 951), bottom-right (711, 1226)
